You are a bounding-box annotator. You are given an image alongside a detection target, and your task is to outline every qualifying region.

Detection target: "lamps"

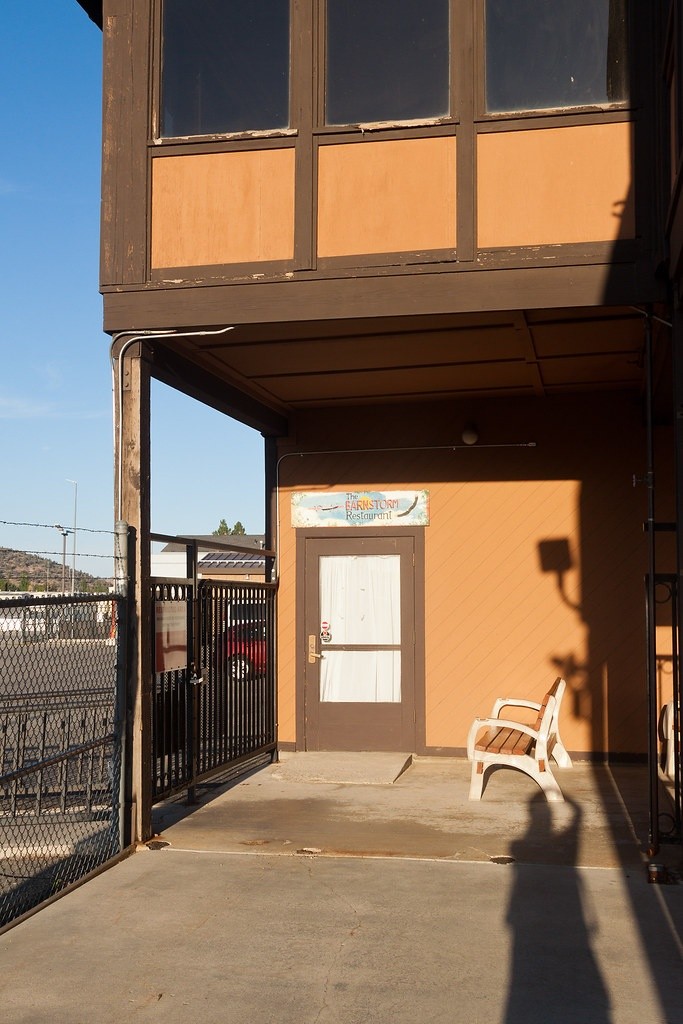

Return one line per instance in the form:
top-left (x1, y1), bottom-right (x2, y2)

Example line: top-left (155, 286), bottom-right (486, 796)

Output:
top-left (462, 425), bottom-right (479, 445)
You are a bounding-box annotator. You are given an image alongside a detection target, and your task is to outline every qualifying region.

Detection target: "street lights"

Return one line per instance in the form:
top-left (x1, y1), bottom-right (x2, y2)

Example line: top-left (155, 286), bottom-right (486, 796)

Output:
top-left (66, 478), bottom-right (78, 596)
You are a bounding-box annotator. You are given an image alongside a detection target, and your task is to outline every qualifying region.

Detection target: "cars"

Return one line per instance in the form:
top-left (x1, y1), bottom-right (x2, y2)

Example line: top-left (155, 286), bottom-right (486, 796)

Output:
top-left (214, 621), bottom-right (266, 683)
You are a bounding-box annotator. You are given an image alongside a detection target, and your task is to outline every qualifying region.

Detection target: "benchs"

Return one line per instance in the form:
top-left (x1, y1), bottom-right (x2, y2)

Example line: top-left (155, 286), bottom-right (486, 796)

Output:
top-left (466, 678), bottom-right (573, 803)
